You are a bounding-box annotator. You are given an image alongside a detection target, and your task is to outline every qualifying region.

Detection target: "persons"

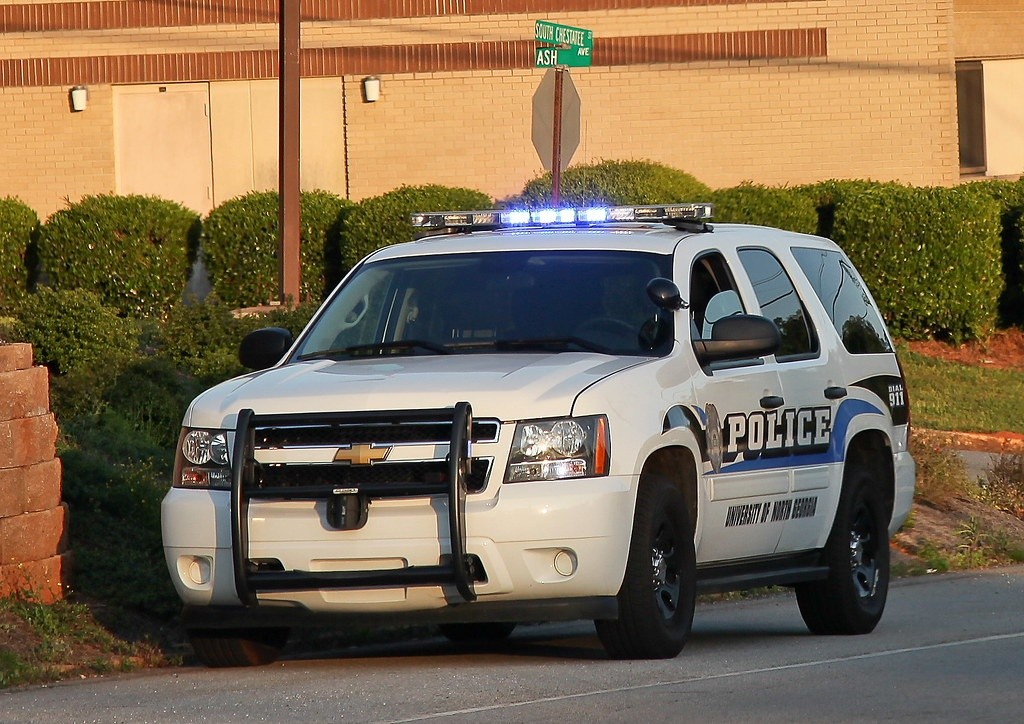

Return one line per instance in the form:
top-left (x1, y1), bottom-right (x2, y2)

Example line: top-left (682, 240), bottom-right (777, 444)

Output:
top-left (599, 258), bottom-right (662, 354)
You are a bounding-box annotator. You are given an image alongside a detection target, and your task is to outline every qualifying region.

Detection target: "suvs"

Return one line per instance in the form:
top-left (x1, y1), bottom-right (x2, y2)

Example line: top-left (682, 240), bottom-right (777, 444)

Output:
top-left (160, 202), bottom-right (915, 659)
